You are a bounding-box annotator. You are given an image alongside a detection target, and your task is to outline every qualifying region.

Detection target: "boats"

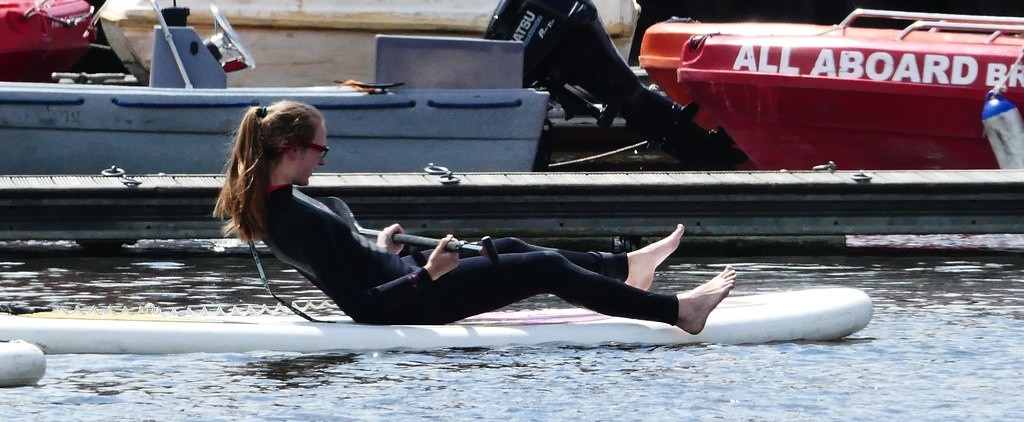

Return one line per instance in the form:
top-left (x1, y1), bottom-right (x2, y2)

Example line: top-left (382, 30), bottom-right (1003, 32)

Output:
top-left (680, 11), bottom-right (1023, 167)
top-left (634, 18), bottom-right (1024, 131)
top-left (0, 0), bottom-right (258, 91)
top-left (0, 30), bottom-right (550, 178)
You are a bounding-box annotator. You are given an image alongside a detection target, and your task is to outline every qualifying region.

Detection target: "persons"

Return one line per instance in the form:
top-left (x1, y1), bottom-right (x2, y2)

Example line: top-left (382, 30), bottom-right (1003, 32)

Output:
top-left (211, 101), bottom-right (738, 335)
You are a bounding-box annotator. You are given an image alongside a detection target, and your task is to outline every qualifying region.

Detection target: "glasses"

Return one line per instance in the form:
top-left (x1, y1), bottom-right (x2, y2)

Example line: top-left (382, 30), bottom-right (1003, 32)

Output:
top-left (300, 142), bottom-right (329, 158)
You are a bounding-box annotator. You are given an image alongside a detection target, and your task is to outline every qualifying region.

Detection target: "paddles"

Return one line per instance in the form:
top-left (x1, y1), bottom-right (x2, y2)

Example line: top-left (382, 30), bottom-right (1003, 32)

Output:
top-left (324, 197), bottom-right (498, 266)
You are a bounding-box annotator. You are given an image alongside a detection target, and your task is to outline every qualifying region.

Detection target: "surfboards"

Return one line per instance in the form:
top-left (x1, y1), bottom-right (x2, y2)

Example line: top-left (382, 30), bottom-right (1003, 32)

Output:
top-left (1, 288), bottom-right (871, 352)
top-left (1, 339), bottom-right (49, 388)
